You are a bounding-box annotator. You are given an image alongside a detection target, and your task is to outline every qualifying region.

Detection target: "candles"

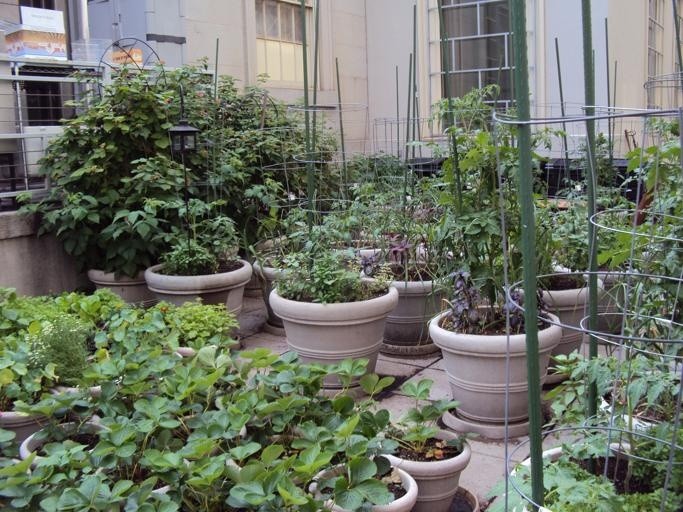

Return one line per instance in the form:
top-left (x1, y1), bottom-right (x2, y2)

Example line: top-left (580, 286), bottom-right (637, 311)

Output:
top-left (141, 364), bottom-right (245, 477)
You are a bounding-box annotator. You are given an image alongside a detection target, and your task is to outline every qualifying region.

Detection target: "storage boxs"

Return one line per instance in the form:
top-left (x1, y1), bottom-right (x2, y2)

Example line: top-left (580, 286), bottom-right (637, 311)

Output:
top-left (0, 6), bottom-right (67, 59)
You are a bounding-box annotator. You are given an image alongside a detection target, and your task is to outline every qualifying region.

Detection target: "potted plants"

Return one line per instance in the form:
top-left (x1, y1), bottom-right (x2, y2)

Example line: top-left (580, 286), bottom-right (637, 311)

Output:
top-left (363, 158), bottom-right (428, 228)
top-left (32, 323), bottom-right (123, 412)
top-left (550, 213), bottom-right (640, 332)
top-left (308, 411), bottom-right (418, 512)
top-left (598, 312), bottom-right (683, 441)
top-left (19, 395), bottom-right (112, 476)
top-left (270, 201), bottom-right (398, 388)
top-left (624, 218), bottom-right (682, 374)
top-left (145, 153), bottom-right (250, 332)
top-left (428, 143), bottom-right (560, 422)
top-left (18, 84), bottom-right (179, 307)
top-left (252, 186), bottom-right (330, 263)
top-left (373, 379), bottom-right (472, 512)
top-left (138, 302), bottom-right (235, 388)
top-left (509, 220), bottom-right (604, 373)
top-left (89, 437), bottom-right (182, 512)
top-left (361, 191), bottom-right (466, 351)
top-left (512, 338), bottom-right (683, 512)
top-left (254, 211), bottom-right (357, 328)
top-left (429, 83), bottom-right (502, 210)
top-left (1, 360), bottom-right (58, 456)
top-left (215, 381), bottom-right (313, 484)
